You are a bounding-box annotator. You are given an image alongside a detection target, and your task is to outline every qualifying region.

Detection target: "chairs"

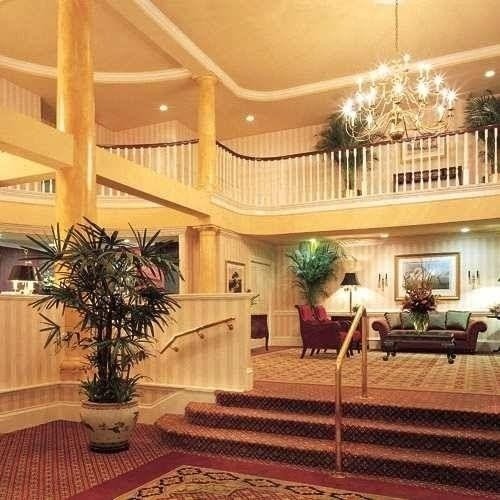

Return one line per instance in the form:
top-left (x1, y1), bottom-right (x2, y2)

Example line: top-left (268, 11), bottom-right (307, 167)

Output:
top-left (294, 303), bottom-right (362, 358)
top-left (251, 314), bottom-right (270, 351)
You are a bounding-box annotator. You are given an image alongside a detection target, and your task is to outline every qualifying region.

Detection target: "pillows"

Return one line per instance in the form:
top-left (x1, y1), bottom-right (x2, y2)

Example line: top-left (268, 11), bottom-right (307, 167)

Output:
top-left (384, 310), bottom-right (472, 330)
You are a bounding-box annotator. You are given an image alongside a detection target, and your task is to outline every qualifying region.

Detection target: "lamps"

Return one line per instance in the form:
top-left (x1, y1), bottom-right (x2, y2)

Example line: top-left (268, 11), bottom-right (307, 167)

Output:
top-left (338, 272), bottom-right (360, 313)
top-left (8, 247), bottom-right (44, 295)
top-left (340, 0), bottom-right (460, 146)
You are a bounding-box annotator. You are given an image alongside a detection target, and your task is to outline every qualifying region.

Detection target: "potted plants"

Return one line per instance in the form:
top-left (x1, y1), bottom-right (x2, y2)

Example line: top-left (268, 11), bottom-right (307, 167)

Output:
top-left (460, 91), bottom-right (500, 182)
top-left (17, 214), bottom-right (187, 455)
top-left (64, 449), bottom-right (498, 500)
top-left (311, 110), bottom-right (380, 198)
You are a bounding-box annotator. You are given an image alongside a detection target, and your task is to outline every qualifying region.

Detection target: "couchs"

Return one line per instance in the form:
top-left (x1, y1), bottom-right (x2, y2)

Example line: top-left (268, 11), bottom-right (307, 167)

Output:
top-left (372, 318), bottom-right (487, 355)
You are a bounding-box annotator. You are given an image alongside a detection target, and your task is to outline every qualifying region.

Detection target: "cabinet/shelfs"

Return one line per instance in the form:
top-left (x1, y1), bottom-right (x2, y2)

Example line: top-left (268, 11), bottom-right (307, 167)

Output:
top-left (330, 313), bottom-right (370, 354)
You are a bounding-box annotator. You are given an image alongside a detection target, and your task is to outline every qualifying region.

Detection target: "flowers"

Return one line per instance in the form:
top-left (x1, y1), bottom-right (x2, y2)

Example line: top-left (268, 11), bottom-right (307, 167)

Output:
top-left (401, 266), bottom-right (437, 330)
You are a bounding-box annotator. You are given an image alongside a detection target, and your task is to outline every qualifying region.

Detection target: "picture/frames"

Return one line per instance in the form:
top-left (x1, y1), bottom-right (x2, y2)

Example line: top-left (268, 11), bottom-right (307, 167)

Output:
top-left (400, 127), bottom-right (448, 163)
top-left (394, 251), bottom-right (461, 301)
top-left (224, 260), bottom-right (247, 293)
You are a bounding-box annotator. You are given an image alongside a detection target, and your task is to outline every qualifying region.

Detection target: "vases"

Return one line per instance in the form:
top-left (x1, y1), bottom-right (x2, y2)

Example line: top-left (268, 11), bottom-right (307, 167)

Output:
top-left (412, 312), bottom-right (430, 335)
